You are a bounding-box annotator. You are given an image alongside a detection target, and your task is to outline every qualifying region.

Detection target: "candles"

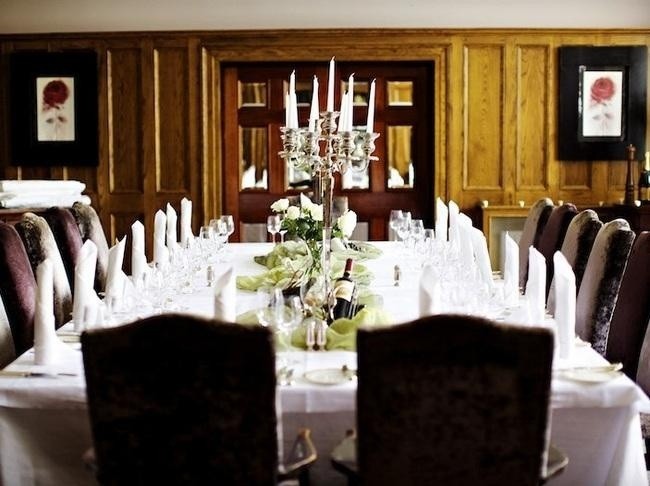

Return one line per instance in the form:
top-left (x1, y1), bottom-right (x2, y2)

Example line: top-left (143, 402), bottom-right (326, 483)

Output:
top-left (290, 68), bottom-right (296, 127)
top-left (344, 92), bottom-right (348, 131)
top-left (366, 75), bottom-right (377, 132)
top-left (313, 75), bottom-right (320, 118)
top-left (309, 77), bottom-right (319, 131)
top-left (348, 72), bottom-right (356, 132)
top-left (285, 91), bottom-right (289, 126)
top-left (326, 55), bottom-right (336, 110)
top-left (339, 89), bottom-right (346, 131)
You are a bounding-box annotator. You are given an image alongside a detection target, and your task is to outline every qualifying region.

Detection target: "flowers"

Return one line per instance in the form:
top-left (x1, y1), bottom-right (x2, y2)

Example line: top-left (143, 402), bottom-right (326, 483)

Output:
top-left (269, 191), bottom-right (357, 272)
top-left (584, 68), bottom-right (622, 135)
top-left (38, 77), bottom-right (73, 141)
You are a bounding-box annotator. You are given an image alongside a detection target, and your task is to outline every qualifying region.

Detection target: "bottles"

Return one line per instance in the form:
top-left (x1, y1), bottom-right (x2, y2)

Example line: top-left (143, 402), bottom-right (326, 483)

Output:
top-left (328, 255), bottom-right (358, 326)
top-left (639, 149), bottom-right (649, 204)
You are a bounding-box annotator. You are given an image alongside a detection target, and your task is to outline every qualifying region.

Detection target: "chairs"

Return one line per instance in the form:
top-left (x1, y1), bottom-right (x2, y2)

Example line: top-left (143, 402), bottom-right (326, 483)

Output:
top-left (79, 312), bottom-right (318, 485)
top-left (0, 201), bottom-right (129, 370)
top-left (517, 197), bottom-right (650, 398)
top-left (331, 311), bottom-right (570, 484)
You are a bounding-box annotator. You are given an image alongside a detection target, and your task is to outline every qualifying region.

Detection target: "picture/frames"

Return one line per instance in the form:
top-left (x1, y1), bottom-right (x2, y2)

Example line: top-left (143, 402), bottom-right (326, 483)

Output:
top-left (0, 46), bottom-right (99, 164)
top-left (557, 44), bottom-right (648, 161)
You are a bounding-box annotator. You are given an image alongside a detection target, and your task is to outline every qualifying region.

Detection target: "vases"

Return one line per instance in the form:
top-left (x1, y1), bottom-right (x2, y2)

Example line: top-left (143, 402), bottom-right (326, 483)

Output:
top-left (301, 226), bottom-right (340, 321)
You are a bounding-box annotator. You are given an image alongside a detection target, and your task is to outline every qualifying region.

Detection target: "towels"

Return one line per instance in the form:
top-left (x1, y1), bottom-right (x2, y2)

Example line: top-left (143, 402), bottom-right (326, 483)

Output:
top-left (1, 194), bottom-right (93, 206)
top-left (435, 196), bottom-right (517, 302)
top-left (211, 266), bottom-right (238, 326)
top-left (132, 195), bottom-right (194, 284)
top-left (419, 263), bottom-right (443, 317)
top-left (241, 160), bottom-right (414, 190)
top-left (0, 178), bottom-right (86, 193)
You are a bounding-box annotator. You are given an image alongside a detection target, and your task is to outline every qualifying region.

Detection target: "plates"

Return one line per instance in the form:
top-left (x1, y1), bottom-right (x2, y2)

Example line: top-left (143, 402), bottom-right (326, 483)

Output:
top-left (558, 364), bottom-right (625, 385)
top-left (302, 365), bottom-right (355, 386)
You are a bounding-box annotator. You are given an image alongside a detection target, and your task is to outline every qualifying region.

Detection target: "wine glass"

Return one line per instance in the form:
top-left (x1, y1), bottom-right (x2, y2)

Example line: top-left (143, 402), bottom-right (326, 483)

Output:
top-left (81, 213), bottom-right (236, 336)
top-left (266, 211), bottom-right (288, 246)
top-left (253, 282), bottom-right (303, 369)
top-left (388, 206), bottom-right (490, 321)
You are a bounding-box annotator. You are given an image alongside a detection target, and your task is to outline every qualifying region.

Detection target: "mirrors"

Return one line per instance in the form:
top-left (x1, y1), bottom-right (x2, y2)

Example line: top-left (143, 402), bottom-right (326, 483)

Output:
top-left (386, 124), bottom-right (414, 191)
top-left (240, 81), bottom-right (266, 105)
top-left (387, 80), bottom-right (413, 106)
top-left (241, 127), bottom-right (267, 188)
